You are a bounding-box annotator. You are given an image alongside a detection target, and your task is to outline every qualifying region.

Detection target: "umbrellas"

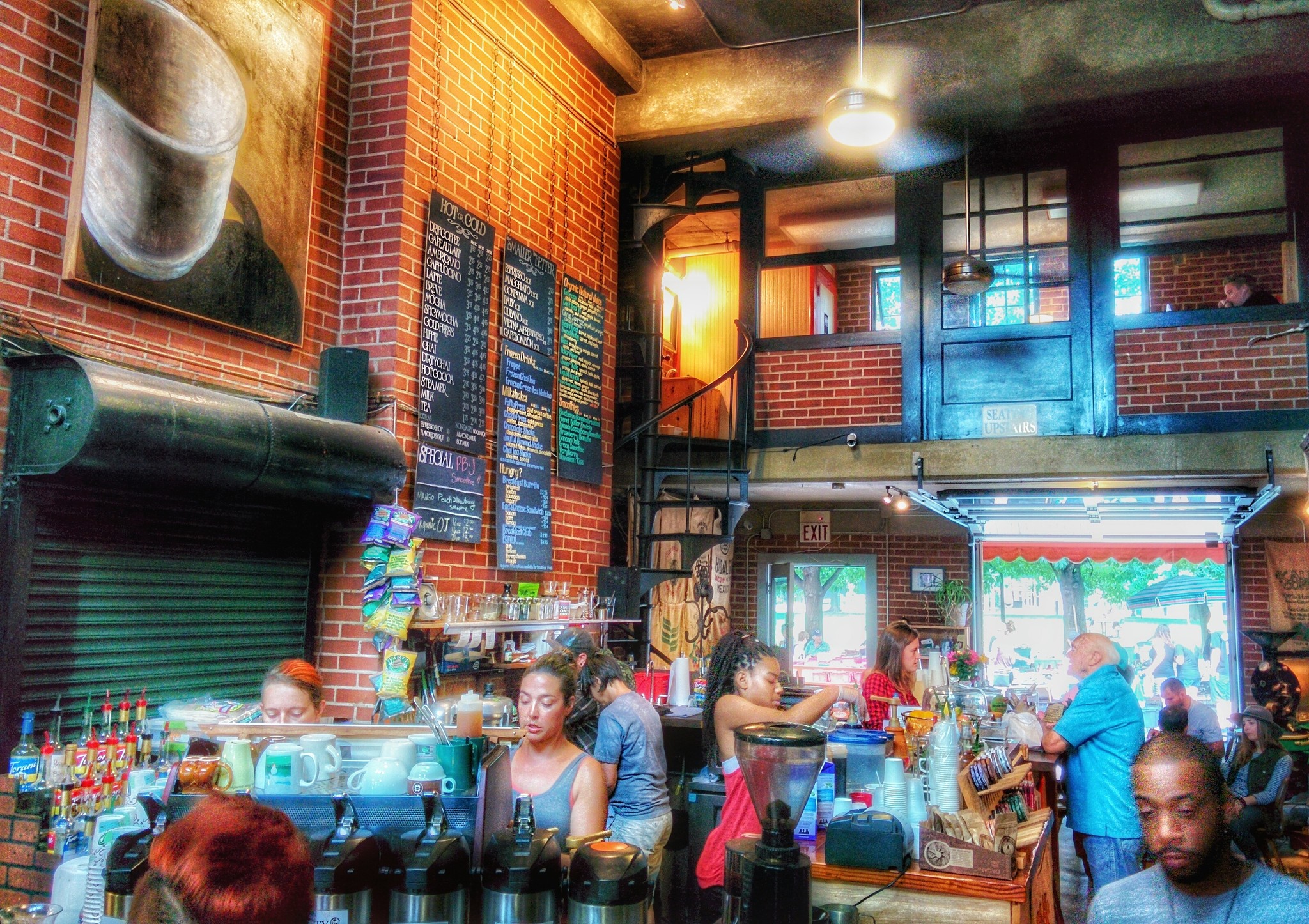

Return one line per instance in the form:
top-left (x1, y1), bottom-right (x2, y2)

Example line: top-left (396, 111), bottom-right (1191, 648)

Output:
top-left (1126, 573), bottom-right (1228, 610)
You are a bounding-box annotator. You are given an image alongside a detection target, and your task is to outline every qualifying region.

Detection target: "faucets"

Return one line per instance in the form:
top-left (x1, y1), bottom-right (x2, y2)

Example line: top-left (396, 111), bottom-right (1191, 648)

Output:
top-left (645, 658), bottom-right (655, 708)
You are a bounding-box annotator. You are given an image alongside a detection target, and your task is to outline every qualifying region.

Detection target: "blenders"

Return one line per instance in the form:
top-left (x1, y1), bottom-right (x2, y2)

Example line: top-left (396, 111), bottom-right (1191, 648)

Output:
top-left (718, 719), bottom-right (829, 923)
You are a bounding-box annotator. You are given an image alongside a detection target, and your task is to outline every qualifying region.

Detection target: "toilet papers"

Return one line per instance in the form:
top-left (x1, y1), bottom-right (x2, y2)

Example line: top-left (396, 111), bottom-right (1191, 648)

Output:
top-left (666, 657), bottom-right (692, 707)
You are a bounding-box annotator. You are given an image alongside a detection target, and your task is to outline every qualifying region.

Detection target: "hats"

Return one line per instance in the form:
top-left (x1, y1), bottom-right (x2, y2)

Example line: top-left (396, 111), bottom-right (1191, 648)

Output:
top-left (1230, 705), bottom-right (1283, 739)
top-left (541, 625), bottom-right (594, 654)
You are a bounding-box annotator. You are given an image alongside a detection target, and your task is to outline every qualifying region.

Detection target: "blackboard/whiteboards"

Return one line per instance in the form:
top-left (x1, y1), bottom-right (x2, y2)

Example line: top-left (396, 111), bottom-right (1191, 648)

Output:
top-left (496, 337), bottom-right (553, 573)
top-left (503, 234), bottom-right (558, 357)
top-left (556, 273), bottom-right (607, 485)
top-left (417, 188), bottom-right (496, 457)
top-left (412, 442), bottom-right (486, 545)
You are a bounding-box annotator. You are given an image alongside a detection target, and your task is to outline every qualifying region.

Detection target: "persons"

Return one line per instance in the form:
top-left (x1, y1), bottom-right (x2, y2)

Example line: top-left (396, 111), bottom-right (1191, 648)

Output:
top-left (579, 651), bottom-right (672, 923)
top-left (1155, 703), bottom-right (1190, 737)
top-left (252, 658), bottom-right (352, 794)
top-left (1080, 733), bottom-right (1309, 922)
top-left (1221, 703), bottom-right (1295, 867)
top-left (1145, 677), bottom-right (1226, 769)
top-left (124, 793), bottom-right (320, 924)
top-left (1036, 633), bottom-right (1147, 902)
top-left (1217, 274), bottom-right (1282, 308)
top-left (540, 627), bottom-right (608, 757)
top-left (693, 629), bottom-right (868, 924)
top-left (1040, 614), bottom-right (1231, 728)
top-left (506, 645), bottom-right (611, 924)
top-left (776, 620), bottom-right (927, 733)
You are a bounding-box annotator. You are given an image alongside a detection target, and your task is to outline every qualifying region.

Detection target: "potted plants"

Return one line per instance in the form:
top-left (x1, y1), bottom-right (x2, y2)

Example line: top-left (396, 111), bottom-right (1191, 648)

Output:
top-left (919, 569), bottom-right (975, 631)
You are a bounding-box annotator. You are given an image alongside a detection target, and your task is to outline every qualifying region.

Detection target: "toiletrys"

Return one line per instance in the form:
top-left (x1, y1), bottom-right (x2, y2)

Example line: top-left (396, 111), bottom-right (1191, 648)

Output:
top-left (871, 692), bottom-right (914, 773)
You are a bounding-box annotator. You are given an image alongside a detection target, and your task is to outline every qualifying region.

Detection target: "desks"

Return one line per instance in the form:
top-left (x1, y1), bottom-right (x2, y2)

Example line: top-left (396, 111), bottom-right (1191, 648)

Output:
top-left (979, 736), bottom-right (1082, 922)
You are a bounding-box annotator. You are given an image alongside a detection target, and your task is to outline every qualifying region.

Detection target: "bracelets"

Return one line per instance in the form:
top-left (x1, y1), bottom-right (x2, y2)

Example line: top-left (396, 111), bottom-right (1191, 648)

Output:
top-left (1237, 797), bottom-right (1248, 808)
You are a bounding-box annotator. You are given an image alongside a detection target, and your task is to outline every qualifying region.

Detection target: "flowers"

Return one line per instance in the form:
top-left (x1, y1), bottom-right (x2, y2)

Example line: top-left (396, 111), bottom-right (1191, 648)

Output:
top-left (947, 648), bottom-right (989, 682)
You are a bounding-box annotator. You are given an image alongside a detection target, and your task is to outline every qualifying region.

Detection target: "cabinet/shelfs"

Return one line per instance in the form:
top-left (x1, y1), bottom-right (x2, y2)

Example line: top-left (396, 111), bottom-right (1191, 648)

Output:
top-left (392, 620), bottom-right (639, 677)
top-left (911, 624), bottom-right (971, 659)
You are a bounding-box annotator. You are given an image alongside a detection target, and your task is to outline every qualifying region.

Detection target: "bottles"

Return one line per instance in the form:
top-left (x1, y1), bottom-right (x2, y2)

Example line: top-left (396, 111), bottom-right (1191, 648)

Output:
top-left (455, 689), bottom-right (483, 737)
top-left (500, 582), bottom-right (514, 619)
top-left (10, 686), bottom-right (169, 859)
top-left (504, 644), bottom-right (513, 663)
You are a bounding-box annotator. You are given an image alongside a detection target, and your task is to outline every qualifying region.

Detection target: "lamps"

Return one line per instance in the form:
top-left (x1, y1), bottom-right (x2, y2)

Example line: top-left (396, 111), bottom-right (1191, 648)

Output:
top-left (940, 9), bottom-right (998, 296)
top-left (818, 0), bottom-right (893, 150)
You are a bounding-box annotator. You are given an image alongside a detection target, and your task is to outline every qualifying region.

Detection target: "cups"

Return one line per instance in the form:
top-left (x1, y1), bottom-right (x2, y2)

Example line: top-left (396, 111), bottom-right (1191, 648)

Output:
top-left (833, 721), bottom-right (959, 860)
top-left (83, 805), bottom-right (135, 922)
top-left (83, 0), bottom-right (249, 283)
top-left (177, 731), bottom-right (487, 795)
top-left (435, 591), bottom-right (616, 623)
top-left (576, 590), bottom-right (599, 620)
top-left (917, 651), bottom-right (950, 689)
top-left (123, 770), bottom-right (167, 826)
top-left (542, 580), bottom-right (570, 596)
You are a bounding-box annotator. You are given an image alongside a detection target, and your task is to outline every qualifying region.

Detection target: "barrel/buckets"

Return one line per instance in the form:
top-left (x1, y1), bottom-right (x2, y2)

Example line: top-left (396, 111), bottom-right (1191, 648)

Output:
top-left (306, 792), bottom-right (653, 924)
top-left (101, 828), bottom-right (155, 923)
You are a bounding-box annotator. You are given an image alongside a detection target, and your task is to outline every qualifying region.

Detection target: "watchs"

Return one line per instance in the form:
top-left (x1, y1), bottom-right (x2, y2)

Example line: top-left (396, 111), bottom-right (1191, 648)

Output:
top-left (1223, 300), bottom-right (1234, 307)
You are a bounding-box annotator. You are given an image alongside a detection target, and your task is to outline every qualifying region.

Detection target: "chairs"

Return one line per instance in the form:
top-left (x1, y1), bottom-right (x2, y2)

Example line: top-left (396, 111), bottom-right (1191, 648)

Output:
top-left (1254, 778), bottom-right (1289, 878)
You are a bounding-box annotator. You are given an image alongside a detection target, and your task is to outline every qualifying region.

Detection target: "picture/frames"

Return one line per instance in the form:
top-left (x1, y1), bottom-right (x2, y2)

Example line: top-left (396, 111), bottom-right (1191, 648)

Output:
top-left (909, 566), bottom-right (946, 593)
